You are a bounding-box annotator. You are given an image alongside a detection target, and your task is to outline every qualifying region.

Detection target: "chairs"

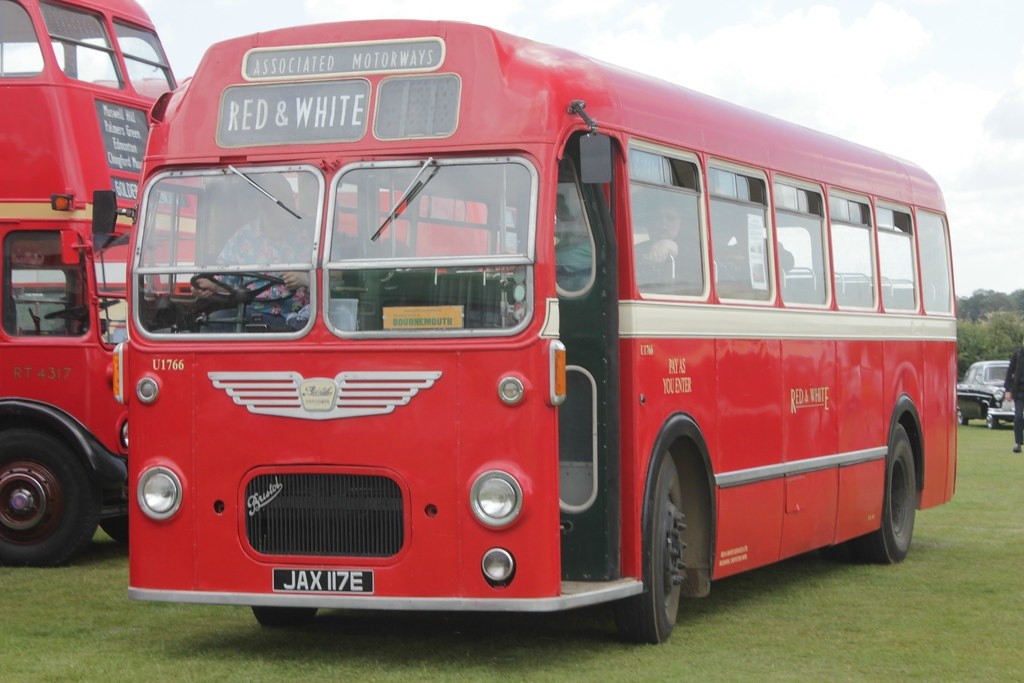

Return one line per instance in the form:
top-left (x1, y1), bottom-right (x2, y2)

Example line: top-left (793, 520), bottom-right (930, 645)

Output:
top-left (639, 256), bottom-right (936, 310)
top-left (339, 235), bottom-right (515, 332)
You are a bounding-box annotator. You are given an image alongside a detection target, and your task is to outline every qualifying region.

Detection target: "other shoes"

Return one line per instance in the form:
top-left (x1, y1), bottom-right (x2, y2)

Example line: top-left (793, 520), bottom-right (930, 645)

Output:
top-left (1013, 443), bottom-right (1021, 452)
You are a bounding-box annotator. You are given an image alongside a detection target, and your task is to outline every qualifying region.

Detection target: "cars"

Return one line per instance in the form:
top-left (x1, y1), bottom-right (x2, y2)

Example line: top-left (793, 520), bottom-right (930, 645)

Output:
top-left (957, 360), bottom-right (1016, 428)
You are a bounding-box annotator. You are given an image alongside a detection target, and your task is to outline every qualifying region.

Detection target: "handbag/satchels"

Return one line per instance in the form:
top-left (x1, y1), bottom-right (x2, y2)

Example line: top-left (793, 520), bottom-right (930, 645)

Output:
top-left (1010, 377), bottom-right (1019, 400)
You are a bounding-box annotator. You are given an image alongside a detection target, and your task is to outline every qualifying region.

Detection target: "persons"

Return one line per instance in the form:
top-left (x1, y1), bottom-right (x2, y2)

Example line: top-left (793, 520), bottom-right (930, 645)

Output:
top-left (524, 169), bottom-right (797, 313)
top-left (1004, 346), bottom-right (1024, 452)
top-left (189, 176), bottom-right (344, 336)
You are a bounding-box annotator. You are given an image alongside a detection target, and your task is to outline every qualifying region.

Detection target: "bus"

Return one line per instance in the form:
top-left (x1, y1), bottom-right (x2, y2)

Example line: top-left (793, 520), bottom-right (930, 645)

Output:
top-left (1, 0), bottom-right (180, 567)
top-left (92, 19), bottom-right (958, 643)
